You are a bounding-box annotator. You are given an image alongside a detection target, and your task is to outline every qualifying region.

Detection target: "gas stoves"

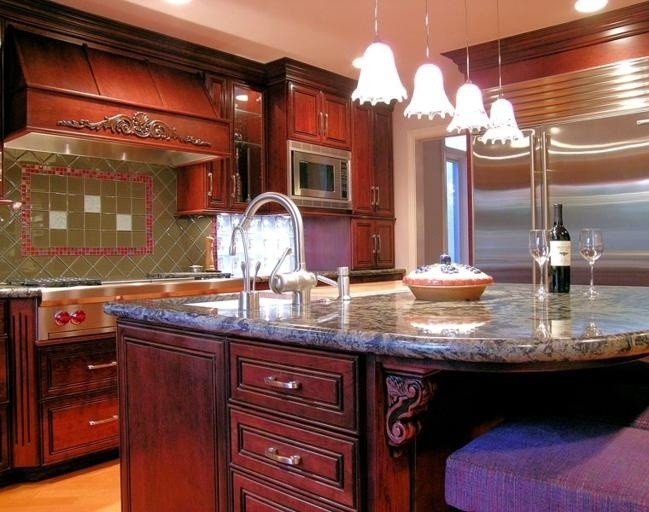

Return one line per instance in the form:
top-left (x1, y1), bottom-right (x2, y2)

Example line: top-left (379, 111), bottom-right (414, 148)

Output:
top-left (7, 276), bottom-right (128, 341)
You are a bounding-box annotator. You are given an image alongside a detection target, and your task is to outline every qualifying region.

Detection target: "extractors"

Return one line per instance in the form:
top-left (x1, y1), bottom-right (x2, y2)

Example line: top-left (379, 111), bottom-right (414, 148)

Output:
top-left (5, 37), bottom-right (235, 167)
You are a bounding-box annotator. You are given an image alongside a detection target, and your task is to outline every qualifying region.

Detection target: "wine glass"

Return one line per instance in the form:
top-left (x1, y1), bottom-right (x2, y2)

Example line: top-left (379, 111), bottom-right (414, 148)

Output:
top-left (534, 304), bottom-right (551, 342)
top-left (579, 228), bottom-right (606, 296)
top-left (527, 229), bottom-right (551, 301)
top-left (581, 301), bottom-right (601, 337)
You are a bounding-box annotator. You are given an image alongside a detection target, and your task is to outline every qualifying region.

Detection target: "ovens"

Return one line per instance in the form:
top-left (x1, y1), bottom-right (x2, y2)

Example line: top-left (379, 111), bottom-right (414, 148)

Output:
top-left (286, 140), bottom-right (352, 200)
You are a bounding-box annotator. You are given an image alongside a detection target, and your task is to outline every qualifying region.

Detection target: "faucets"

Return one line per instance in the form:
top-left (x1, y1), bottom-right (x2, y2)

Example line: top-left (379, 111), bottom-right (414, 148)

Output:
top-left (230, 226), bottom-right (251, 294)
top-left (238, 192), bottom-right (319, 305)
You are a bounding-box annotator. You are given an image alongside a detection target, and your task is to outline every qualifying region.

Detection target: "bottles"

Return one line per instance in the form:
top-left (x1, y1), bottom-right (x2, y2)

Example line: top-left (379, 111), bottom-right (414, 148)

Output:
top-left (551, 296), bottom-right (572, 340)
top-left (549, 201), bottom-right (574, 295)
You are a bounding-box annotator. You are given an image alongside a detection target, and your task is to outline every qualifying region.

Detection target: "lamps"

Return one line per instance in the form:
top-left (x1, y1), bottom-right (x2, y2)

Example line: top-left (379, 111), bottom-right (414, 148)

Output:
top-left (350, 0), bottom-right (525, 149)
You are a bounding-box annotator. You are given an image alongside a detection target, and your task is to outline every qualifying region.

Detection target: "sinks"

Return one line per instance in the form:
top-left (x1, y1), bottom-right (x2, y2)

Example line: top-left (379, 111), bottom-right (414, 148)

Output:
top-left (186, 298), bottom-right (291, 314)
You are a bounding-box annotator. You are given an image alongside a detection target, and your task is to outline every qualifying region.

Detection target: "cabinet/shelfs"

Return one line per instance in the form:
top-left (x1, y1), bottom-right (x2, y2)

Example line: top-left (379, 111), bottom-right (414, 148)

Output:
top-left (177, 74), bottom-right (267, 216)
top-left (229, 335), bottom-right (365, 512)
top-left (36, 333), bottom-right (120, 468)
top-left (250, 83), bottom-right (397, 269)
